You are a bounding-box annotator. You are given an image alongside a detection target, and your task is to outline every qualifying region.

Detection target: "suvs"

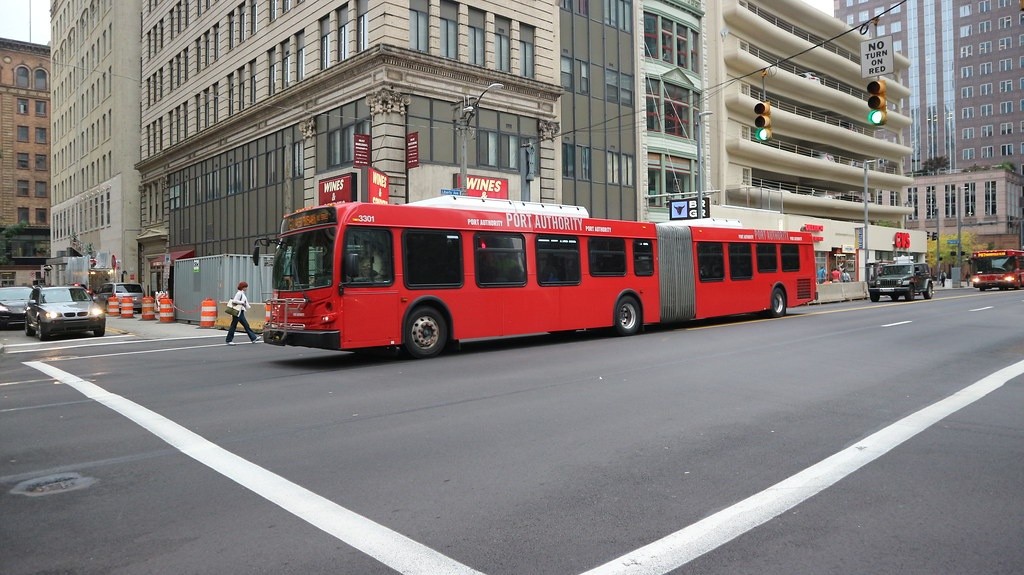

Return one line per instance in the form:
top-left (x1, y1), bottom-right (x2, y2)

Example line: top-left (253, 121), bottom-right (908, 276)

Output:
top-left (24, 284), bottom-right (106, 342)
top-left (869, 263), bottom-right (934, 303)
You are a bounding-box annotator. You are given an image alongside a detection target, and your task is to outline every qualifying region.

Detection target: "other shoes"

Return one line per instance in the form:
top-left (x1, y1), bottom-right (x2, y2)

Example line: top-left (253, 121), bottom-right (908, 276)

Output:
top-left (252, 336), bottom-right (262, 343)
top-left (228, 341), bottom-right (236, 346)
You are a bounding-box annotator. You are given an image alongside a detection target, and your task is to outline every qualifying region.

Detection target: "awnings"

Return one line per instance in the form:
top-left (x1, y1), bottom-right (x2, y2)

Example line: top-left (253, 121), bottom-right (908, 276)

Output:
top-left (151, 249), bottom-right (195, 266)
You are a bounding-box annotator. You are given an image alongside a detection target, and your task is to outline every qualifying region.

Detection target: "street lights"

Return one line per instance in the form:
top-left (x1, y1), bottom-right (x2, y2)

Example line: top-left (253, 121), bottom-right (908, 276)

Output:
top-left (696, 110), bottom-right (714, 219)
top-left (958, 186), bottom-right (968, 267)
top-left (934, 207), bottom-right (940, 285)
top-left (458, 83), bottom-right (504, 196)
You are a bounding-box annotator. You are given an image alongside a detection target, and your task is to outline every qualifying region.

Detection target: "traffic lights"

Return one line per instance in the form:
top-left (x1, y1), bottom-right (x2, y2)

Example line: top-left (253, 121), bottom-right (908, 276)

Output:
top-left (754, 102), bottom-right (773, 141)
top-left (867, 80), bottom-right (887, 126)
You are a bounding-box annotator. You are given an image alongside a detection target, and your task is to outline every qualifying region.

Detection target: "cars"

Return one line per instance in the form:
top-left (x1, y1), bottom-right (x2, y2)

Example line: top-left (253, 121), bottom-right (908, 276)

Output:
top-left (92, 283), bottom-right (144, 314)
top-left (0, 287), bottom-right (34, 331)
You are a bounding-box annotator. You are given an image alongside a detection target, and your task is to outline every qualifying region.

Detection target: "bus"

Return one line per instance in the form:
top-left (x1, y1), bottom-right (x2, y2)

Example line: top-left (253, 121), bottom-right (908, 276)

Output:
top-left (252, 195), bottom-right (819, 358)
top-left (969, 249), bottom-right (1024, 292)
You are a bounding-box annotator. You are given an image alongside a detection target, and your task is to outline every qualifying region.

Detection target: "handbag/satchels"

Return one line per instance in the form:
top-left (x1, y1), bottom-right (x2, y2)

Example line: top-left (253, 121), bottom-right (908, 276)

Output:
top-left (225, 293), bottom-right (243, 317)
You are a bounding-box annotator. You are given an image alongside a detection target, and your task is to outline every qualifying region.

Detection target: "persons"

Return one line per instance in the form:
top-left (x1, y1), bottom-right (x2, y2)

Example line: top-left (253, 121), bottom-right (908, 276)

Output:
top-left (966, 271), bottom-right (970, 286)
top-left (352, 259), bottom-right (380, 280)
top-left (226, 281), bottom-right (261, 345)
top-left (818, 266), bottom-right (827, 283)
top-left (831, 267), bottom-right (851, 282)
top-left (940, 270), bottom-right (946, 287)
